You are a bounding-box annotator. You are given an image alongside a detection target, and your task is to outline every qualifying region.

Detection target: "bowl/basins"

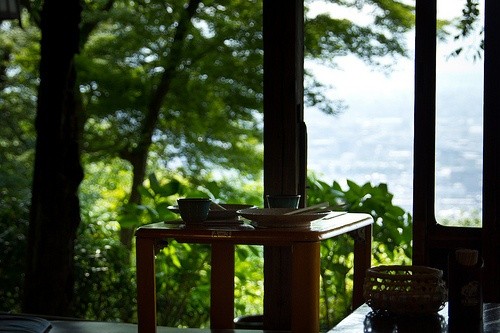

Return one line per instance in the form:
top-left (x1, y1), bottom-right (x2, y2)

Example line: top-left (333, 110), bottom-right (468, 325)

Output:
top-left (177, 197), bottom-right (212, 225)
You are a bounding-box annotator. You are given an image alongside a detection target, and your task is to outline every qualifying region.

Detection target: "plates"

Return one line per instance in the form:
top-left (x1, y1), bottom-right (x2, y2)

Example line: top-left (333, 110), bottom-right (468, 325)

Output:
top-left (0, 312), bottom-right (55, 333)
top-left (236, 207), bottom-right (332, 227)
top-left (166, 203), bottom-right (256, 221)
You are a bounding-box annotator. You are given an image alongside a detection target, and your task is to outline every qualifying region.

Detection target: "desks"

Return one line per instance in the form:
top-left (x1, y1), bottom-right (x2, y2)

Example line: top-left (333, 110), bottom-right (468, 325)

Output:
top-left (135, 209), bottom-right (375, 333)
